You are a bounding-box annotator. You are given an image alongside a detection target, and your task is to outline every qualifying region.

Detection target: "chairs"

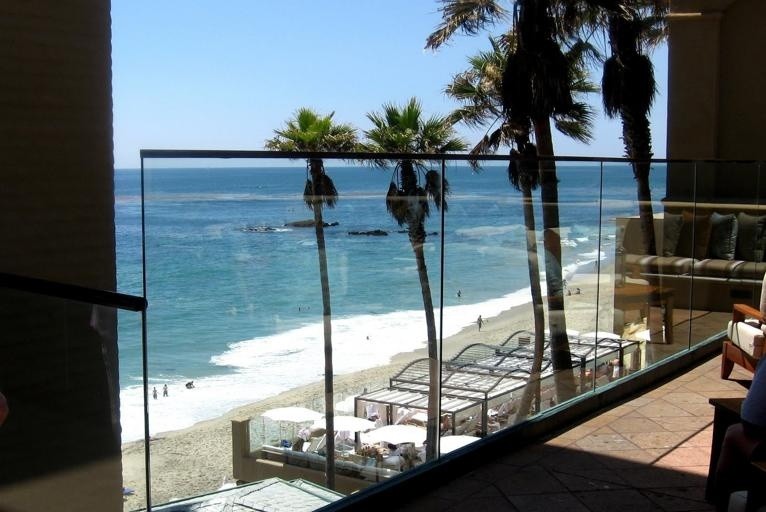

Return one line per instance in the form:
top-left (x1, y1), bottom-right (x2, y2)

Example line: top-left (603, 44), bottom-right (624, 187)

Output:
top-left (719, 258), bottom-right (766, 385)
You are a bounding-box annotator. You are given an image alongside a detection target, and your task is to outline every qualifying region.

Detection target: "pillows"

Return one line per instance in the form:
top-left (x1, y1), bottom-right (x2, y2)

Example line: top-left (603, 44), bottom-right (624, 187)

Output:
top-left (663, 210), bottom-right (766, 263)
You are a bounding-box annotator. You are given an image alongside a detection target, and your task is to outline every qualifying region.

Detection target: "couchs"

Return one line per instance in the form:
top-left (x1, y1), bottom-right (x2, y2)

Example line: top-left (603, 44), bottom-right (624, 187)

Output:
top-left (611, 203), bottom-right (766, 318)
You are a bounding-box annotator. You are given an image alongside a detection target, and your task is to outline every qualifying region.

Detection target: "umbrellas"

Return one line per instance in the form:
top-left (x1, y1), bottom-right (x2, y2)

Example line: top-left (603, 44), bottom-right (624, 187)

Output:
top-left (259, 405), bottom-right (324, 445)
top-left (357, 423), bottom-right (427, 474)
top-left (439, 435), bottom-right (481, 456)
top-left (309, 414), bottom-right (376, 459)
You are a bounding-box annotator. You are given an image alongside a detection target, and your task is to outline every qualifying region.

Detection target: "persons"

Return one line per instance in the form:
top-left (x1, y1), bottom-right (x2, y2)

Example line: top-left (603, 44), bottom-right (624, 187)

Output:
top-left (473, 429), bottom-right (481, 437)
top-left (386, 444), bottom-right (410, 459)
top-left (563, 278), bottom-right (567, 289)
top-left (184, 380), bottom-right (193, 390)
top-left (290, 437), bottom-right (305, 454)
top-left (604, 359), bottom-right (619, 377)
top-left (476, 314), bottom-right (483, 332)
top-left (365, 335), bottom-right (369, 341)
top-left (593, 259), bottom-right (597, 272)
top-left (317, 445), bottom-right (326, 458)
top-left (456, 289), bottom-right (461, 297)
top-left (162, 383), bottom-right (168, 397)
top-left (297, 306), bottom-right (300, 312)
top-left (565, 289), bottom-right (571, 296)
top-left (573, 288), bottom-right (580, 294)
top-left (370, 414), bottom-right (383, 429)
top-left (441, 414), bottom-right (452, 432)
top-left (151, 386), bottom-right (158, 400)
